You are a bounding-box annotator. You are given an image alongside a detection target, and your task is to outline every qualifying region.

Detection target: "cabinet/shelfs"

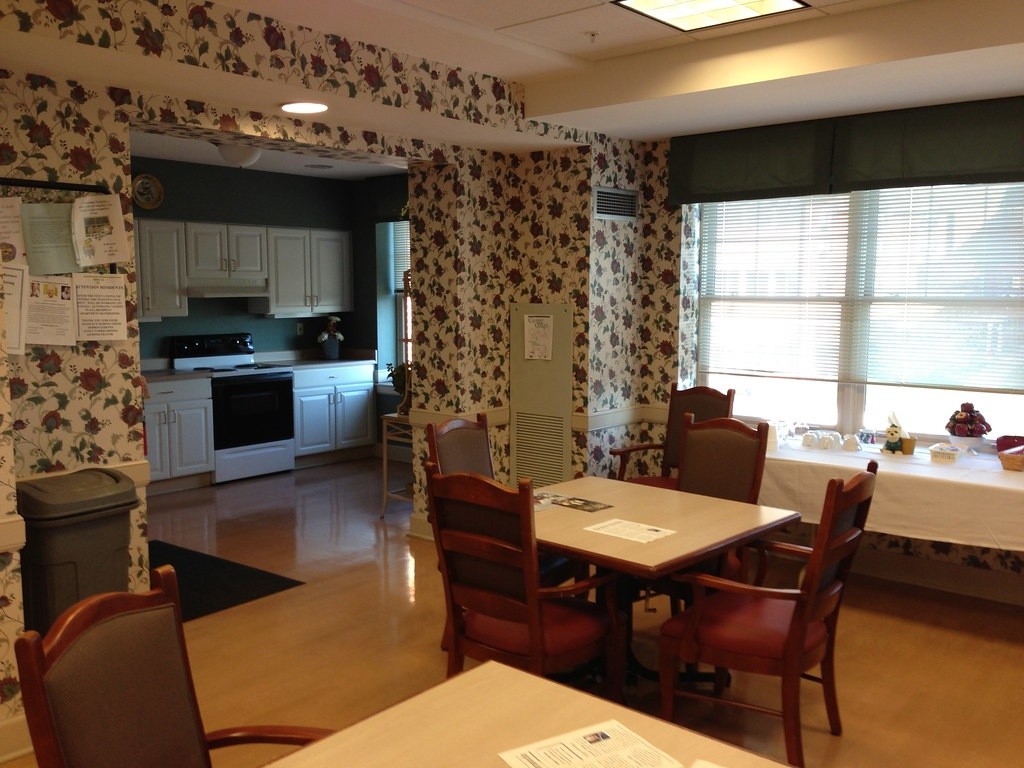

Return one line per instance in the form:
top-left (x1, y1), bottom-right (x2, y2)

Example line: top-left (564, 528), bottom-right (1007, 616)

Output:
top-left (133, 218), bottom-right (355, 322)
top-left (144, 378), bottom-right (215, 496)
top-left (380, 268), bottom-right (414, 518)
top-left (292, 365), bottom-right (376, 469)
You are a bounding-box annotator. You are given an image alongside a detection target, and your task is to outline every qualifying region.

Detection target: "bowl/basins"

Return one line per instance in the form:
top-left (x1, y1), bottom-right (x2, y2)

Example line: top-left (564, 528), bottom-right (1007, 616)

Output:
top-left (949, 434), bottom-right (985, 448)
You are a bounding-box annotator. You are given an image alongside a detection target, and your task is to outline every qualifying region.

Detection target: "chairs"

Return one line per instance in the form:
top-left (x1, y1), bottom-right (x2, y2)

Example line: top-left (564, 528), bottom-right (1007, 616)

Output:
top-left (13, 565), bottom-right (340, 768)
top-left (609, 383), bottom-right (735, 490)
top-left (658, 459), bottom-right (877, 768)
top-left (425, 462), bottom-right (628, 706)
top-left (428, 411), bottom-right (590, 651)
top-left (634, 412), bottom-right (769, 672)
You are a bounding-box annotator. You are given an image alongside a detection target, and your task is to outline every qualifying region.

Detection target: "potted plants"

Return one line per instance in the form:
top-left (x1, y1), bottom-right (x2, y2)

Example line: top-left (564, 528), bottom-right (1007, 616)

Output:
top-left (386, 363), bottom-right (412, 414)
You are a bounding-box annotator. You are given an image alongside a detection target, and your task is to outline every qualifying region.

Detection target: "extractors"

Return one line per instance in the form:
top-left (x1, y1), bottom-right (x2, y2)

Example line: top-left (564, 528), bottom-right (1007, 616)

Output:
top-left (187, 277), bottom-right (272, 298)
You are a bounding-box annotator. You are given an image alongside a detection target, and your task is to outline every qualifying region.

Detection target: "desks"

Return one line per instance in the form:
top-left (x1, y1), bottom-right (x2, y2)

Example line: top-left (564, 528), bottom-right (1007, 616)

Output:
top-left (532, 476), bottom-right (801, 683)
top-left (757, 438), bottom-right (1024, 552)
top-left (254, 660), bottom-right (802, 768)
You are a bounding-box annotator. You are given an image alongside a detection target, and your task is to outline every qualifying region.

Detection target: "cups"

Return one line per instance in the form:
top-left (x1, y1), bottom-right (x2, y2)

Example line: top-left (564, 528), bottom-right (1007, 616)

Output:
top-left (900, 436), bottom-right (918, 455)
top-left (802, 430), bottom-right (862, 452)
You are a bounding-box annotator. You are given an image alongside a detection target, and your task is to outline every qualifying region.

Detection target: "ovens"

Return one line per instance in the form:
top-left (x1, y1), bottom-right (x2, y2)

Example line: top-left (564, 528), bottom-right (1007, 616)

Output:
top-left (210, 372), bottom-right (296, 485)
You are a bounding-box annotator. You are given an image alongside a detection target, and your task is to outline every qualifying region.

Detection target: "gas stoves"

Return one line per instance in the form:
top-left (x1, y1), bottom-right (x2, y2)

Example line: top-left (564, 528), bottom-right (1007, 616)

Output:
top-left (162, 332), bottom-right (294, 378)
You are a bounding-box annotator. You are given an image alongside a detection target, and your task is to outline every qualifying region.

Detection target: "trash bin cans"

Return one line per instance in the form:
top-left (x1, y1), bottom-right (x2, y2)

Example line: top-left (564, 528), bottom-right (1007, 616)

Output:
top-left (15, 466), bottom-right (141, 641)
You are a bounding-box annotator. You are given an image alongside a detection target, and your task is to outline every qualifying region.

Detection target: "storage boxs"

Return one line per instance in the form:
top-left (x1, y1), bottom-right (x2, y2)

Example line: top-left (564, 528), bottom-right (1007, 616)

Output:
top-left (997, 445), bottom-right (1024, 471)
top-left (929, 442), bottom-right (968, 462)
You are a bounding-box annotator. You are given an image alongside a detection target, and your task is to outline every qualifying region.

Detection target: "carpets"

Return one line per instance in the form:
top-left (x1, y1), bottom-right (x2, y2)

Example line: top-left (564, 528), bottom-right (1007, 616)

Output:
top-left (148, 539), bottom-right (305, 623)
